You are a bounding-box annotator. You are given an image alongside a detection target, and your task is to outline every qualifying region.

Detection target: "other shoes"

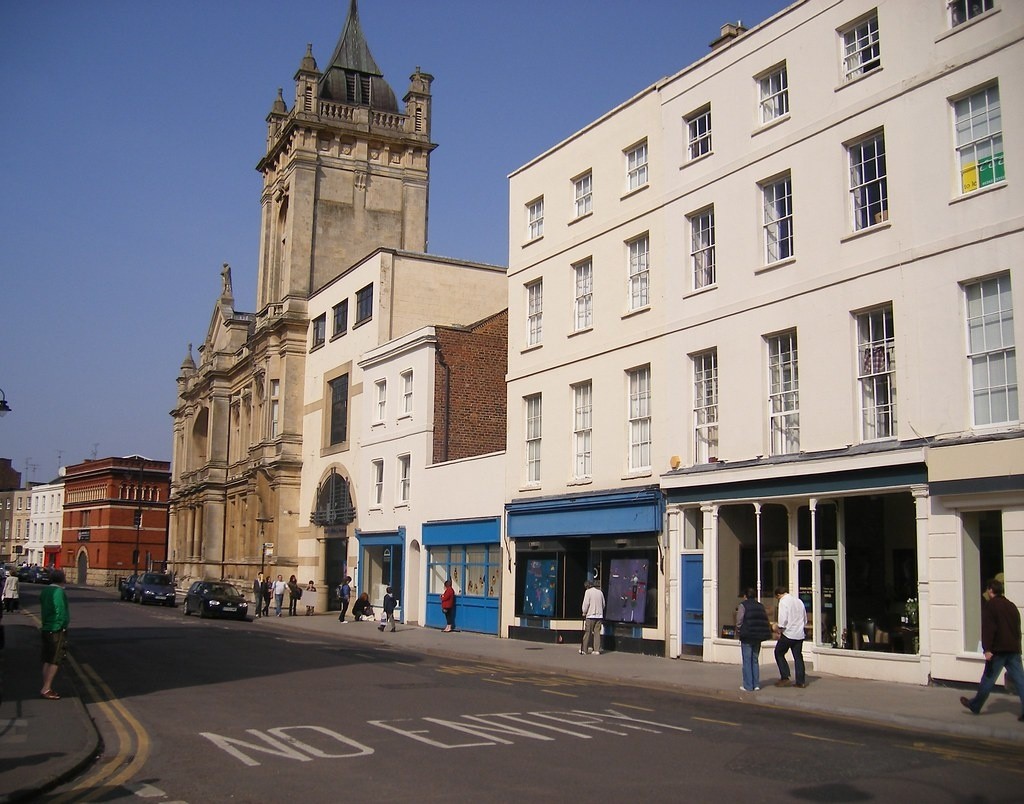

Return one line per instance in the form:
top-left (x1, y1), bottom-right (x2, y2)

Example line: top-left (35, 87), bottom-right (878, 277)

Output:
top-left (390, 627), bottom-right (396, 632)
top-left (441, 629), bottom-right (453, 632)
top-left (255, 611), bottom-right (300, 619)
top-left (754, 687), bottom-right (761, 691)
top-left (578, 650), bottom-right (587, 655)
top-left (39, 690), bottom-right (61, 700)
top-left (792, 683), bottom-right (806, 688)
top-left (591, 651), bottom-right (599, 656)
top-left (774, 677), bottom-right (791, 687)
top-left (378, 626), bottom-right (385, 632)
top-left (960, 696), bottom-right (978, 714)
top-left (740, 686), bottom-right (747, 691)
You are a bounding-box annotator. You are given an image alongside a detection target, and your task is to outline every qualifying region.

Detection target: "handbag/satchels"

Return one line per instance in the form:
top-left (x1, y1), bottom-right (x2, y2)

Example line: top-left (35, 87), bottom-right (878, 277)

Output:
top-left (380, 612), bottom-right (388, 625)
top-left (1003, 671), bottom-right (1020, 695)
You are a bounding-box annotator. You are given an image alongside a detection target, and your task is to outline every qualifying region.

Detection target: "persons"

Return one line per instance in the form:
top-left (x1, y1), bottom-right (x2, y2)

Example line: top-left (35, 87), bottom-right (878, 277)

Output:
top-left (305, 580), bottom-right (316, 616)
top-left (960, 579), bottom-right (1024, 722)
top-left (253, 571), bottom-right (299, 619)
top-left (579, 580), bottom-right (606, 655)
top-left (377, 587), bottom-right (397, 632)
top-left (441, 580), bottom-right (455, 632)
top-left (2, 569), bottom-right (20, 613)
top-left (18, 560), bottom-right (67, 584)
top-left (39, 569), bottom-right (71, 701)
top-left (123, 573), bottom-right (138, 600)
top-left (734, 587), bottom-right (772, 692)
top-left (774, 586), bottom-right (808, 688)
top-left (337, 576), bottom-right (352, 624)
top-left (352, 592), bottom-right (371, 621)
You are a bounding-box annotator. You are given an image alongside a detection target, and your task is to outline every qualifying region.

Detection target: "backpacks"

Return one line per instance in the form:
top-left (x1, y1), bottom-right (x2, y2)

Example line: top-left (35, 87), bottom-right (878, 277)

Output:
top-left (387, 596), bottom-right (397, 609)
top-left (335, 584), bottom-right (343, 602)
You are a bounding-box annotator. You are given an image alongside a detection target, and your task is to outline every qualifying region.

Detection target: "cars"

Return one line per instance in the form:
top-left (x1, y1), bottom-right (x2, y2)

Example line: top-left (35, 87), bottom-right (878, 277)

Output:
top-left (0, 563), bottom-right (51, 584)
top-left (183, 581), bottom-right (248, 621)
top-left (134, 573), bottom-right (178, 607)
top-left (120, 574), bottom-right (139, 602)
